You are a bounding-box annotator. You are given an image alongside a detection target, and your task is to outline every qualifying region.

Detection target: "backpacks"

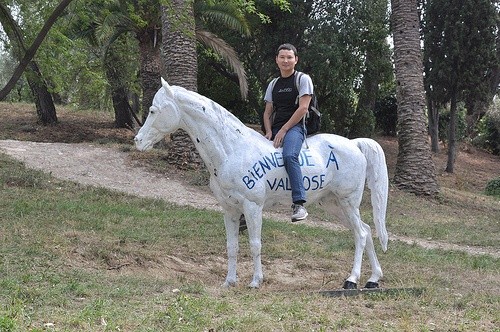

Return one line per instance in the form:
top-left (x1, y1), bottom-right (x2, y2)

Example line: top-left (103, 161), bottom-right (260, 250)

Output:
top-left (295, 73), bottom-right (322, 134)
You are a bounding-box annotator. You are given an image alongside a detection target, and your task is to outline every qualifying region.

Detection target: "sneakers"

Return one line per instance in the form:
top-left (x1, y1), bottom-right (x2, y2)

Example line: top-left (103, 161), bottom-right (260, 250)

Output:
top-left (291, 204), bottom-right (309, 221)
top-left (240, 216), bottom-right (247, 231)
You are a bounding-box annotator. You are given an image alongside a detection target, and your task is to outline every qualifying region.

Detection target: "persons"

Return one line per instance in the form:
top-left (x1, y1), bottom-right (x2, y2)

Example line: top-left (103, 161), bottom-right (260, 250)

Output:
top-left (239, 44), bottom-right (314, 232)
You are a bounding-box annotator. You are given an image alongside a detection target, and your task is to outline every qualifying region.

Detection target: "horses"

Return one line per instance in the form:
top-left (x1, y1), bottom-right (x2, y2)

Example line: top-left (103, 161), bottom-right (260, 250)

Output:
top-left (135, 77), bottom-right (390, 289)
